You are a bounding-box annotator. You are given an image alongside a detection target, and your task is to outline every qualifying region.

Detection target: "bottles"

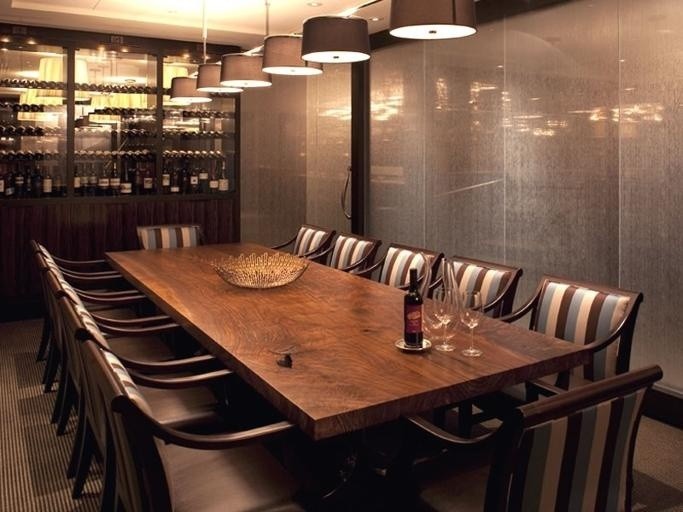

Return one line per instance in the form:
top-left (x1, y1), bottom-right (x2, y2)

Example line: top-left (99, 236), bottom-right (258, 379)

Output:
top-left (218, 157), bottom-right (229, 192)
top-left (73, 163), bottom-right (81, 195)
top-left (0, 150), bottom-right (222, 159)
top-left (143, 162), bottom-right (152, 194)
top-left (15, 171), bottom-right (24, 197)
top-left (0, 174), bottom-right (5, 197)
top-left (90, 162), bottom-right (97, 196)
top-left (32, 164), bottom-right (42, 198)
top-left (97, 108), bottom-right (233, 118)
top-left (0, 126), bottom-right (233, 137)
top-left (43, 167), bottom-right (52, 196)
top-left (210, 170), bottom-right (218, 193)
top-left (82, 163), bottom-right (88, 196)
top-left (162, 163), bottom-right (170, 194)
top-left (0, 78), bottom-right (171, 94)
top-left (199, 166), bottom-right (208, 192)
top-left (170, 167), bottom-right (179, 194)
top-left (120, 162), bottom-right (132, 194)
top-left (5, 167), bottom-right (14, 199)
top-left (98, 161), bottom-right (109, 195)
top-left (52, 175), bottom-right (62, 197)
top-left (190, 168), bottom-right (198, 192)
top-left (0, 103), bottom-right (47, 112)
top-left (25, 167), bottom-right (32, 194)
top-left (109, 161), bottom-right (120, 195)
top-left (134, 163), bottom-right (142, 194)
top-left (182, 169), bottom-right (188, 195)
top-left (403, 269), bottom-right (424, 348)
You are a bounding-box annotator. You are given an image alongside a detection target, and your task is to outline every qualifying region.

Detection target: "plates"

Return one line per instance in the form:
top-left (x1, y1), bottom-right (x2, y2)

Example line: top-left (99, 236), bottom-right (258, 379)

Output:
top-left (212, 253), bottom-right (309, 289)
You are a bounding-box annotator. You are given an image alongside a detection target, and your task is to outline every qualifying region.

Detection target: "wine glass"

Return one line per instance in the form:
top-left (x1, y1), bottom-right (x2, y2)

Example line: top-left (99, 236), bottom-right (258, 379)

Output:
top-left (433, 288), bottom-right (458, 352)
top-left (461, 292), bottom-right (483, 357)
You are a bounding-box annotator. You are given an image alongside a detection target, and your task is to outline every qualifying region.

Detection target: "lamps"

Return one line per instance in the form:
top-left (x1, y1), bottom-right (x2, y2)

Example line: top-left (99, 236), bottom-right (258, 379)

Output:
top-left (219, 53), bottom-right (272, 88)
top-left (196, 63), bottom-right (244, 93)
top-left (387, 0), bottom-right (478, 41)
top-left (260, 34), bottom-right (323, 76)
top-left (170, 77), bottom-right (213, 103)
top-left (299, 15), bottom-right (371, 64)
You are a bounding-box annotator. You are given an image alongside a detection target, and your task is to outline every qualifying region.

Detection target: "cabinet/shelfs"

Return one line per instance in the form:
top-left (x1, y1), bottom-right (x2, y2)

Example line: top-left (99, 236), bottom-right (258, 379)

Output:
top-left (0, 22), bottom-right (242, 323)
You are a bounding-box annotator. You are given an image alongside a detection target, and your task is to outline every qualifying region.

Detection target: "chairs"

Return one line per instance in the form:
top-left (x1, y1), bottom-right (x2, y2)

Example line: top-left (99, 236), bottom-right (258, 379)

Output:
top-left (456, 273), bottom-right (643, 440)
top-left (55, 296), bottom-right (234, 480)
top-left (45, 270), bottom-right (171, 479)
top-left (351, 243), bottom-right (444, 291)
top-left (136, 224), bottom-right (202, 250)
top-left (77, 339), bottom-right (302, 512)
top-left (35, 252), bottom-right (139, 425)
top-left (426, 255), bottom-right (523, 429)
top-left (270, 223), bottom-right (336, 258)
top-left (418, 364), bottom-right (664, 512)
top-left (29, 239), bottom-right (120, 385)
top-left (306, 231), bottom-right (382, 272)
